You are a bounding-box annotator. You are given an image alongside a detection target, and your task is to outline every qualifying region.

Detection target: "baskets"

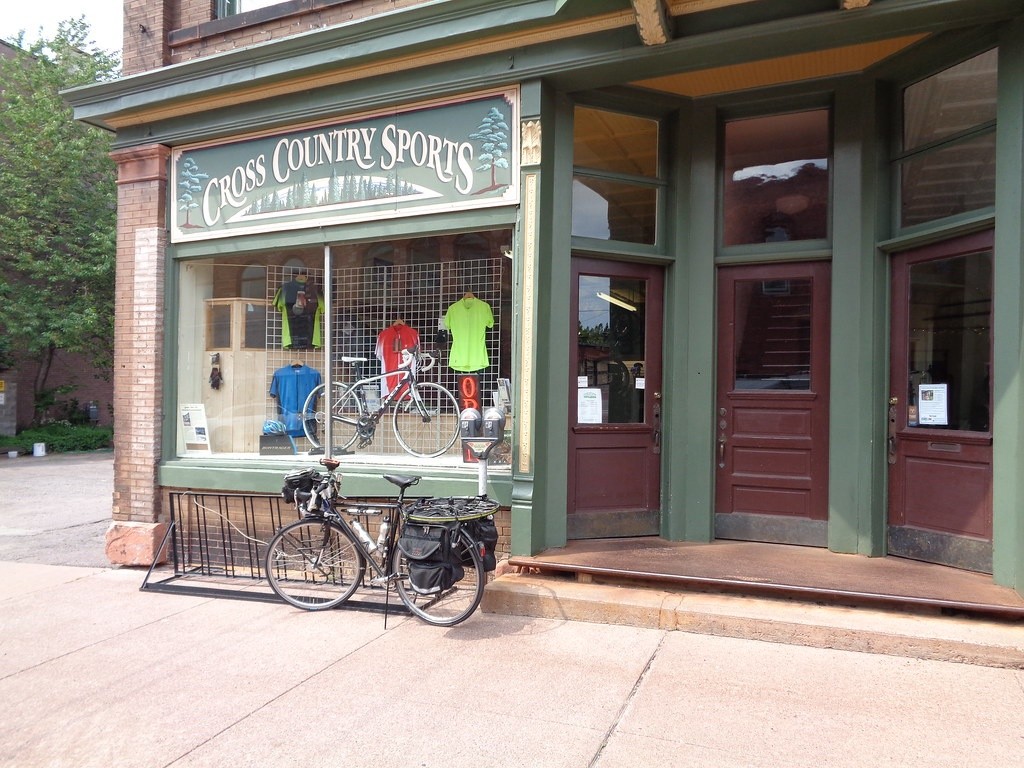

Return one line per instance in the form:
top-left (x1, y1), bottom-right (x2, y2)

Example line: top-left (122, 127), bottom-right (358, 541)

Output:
top-left (297, 474), bottom-right (331, 518)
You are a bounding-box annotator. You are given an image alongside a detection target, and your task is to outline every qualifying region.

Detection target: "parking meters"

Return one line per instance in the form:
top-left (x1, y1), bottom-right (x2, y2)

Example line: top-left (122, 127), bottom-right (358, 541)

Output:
top-left (460, 409), bottom-right (506, 496)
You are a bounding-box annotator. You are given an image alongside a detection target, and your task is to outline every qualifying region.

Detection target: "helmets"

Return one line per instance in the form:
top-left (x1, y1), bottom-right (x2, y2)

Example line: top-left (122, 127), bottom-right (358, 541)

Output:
top-left (262, 420), bottom-right (287, 436)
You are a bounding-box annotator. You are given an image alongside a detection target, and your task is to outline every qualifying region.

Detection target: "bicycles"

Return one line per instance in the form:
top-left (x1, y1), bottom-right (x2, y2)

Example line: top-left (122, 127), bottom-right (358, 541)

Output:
top-left (579, 317), bottom-right (630, 396)
top-left (303, 346), bottom-right (460, 458)
top-left (263, 458), bottom-right (500, 630)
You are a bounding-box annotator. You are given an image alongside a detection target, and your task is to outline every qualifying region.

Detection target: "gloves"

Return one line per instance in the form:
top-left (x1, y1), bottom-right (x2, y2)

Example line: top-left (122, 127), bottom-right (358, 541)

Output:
top-left (209, 353), bottom-right (223, 390)
top-left (434, 329), bottom-right (448, 349)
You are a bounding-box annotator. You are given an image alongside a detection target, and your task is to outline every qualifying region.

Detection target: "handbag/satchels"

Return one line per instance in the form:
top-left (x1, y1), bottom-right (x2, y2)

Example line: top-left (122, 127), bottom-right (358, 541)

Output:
top-left (397, 521), bottom-right (464, 595)
top-left (282, 467), bottom-right (323, 505)
top-left (462, 515), bottom-right (498, 571)
